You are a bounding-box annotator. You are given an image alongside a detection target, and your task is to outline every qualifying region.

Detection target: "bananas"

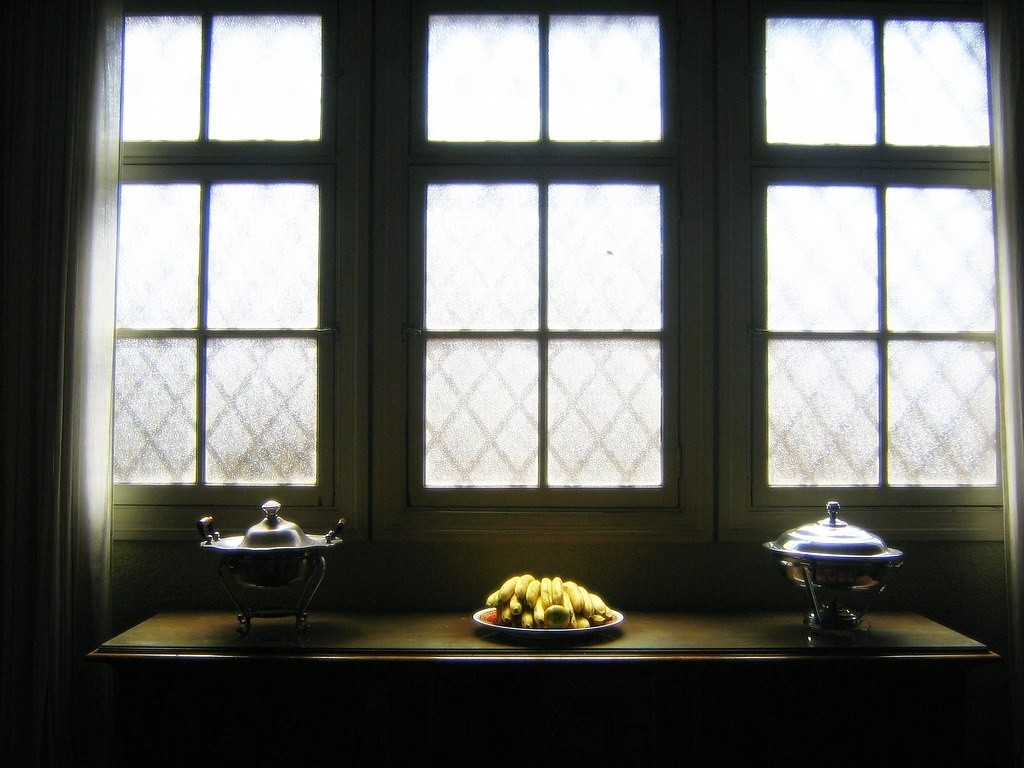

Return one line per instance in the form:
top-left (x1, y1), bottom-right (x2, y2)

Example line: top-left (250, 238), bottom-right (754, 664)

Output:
top-left (484, 574), bottom-right (612, 629)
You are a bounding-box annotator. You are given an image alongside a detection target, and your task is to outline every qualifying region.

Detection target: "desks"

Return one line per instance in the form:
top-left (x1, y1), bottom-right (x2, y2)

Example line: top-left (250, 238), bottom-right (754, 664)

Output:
top-left (83, 614), bottom-right (1003, 768)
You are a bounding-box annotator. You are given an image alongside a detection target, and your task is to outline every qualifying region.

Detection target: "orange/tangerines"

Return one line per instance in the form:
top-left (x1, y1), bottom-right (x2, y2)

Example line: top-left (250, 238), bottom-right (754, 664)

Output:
top-left (544, 606), bottom-right (570, 628)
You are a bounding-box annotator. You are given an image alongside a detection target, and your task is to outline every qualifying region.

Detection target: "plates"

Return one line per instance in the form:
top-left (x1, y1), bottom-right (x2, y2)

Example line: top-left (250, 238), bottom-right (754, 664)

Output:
top-left (473, 608), bottom-right (623, 634)
